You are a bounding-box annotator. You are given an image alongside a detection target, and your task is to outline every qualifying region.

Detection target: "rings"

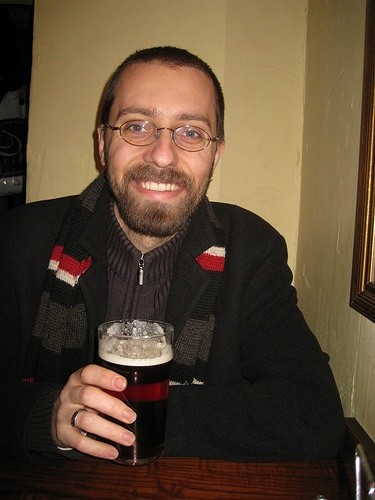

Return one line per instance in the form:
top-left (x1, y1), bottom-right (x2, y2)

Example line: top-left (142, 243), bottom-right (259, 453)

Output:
top-left (71, 408), bottom-right (87, 427)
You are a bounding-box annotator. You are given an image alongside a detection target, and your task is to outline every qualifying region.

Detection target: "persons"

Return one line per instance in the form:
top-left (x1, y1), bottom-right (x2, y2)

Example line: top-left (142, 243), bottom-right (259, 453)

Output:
top-left (1, 46), bottom-right (345, 461)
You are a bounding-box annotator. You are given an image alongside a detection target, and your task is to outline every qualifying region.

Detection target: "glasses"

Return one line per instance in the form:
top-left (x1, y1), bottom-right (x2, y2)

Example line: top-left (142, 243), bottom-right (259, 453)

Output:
top-left (104, 119), bottom-right (221, 152)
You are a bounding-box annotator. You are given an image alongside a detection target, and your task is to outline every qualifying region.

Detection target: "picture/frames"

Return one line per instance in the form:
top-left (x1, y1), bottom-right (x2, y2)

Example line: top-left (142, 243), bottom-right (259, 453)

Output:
top-left (349, 0), bottom-right (375, 324)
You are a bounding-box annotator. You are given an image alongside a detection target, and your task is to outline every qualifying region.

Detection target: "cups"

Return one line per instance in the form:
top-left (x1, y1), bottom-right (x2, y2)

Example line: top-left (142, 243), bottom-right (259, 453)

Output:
top-left (98, 318), bottom-right (175, 467)
